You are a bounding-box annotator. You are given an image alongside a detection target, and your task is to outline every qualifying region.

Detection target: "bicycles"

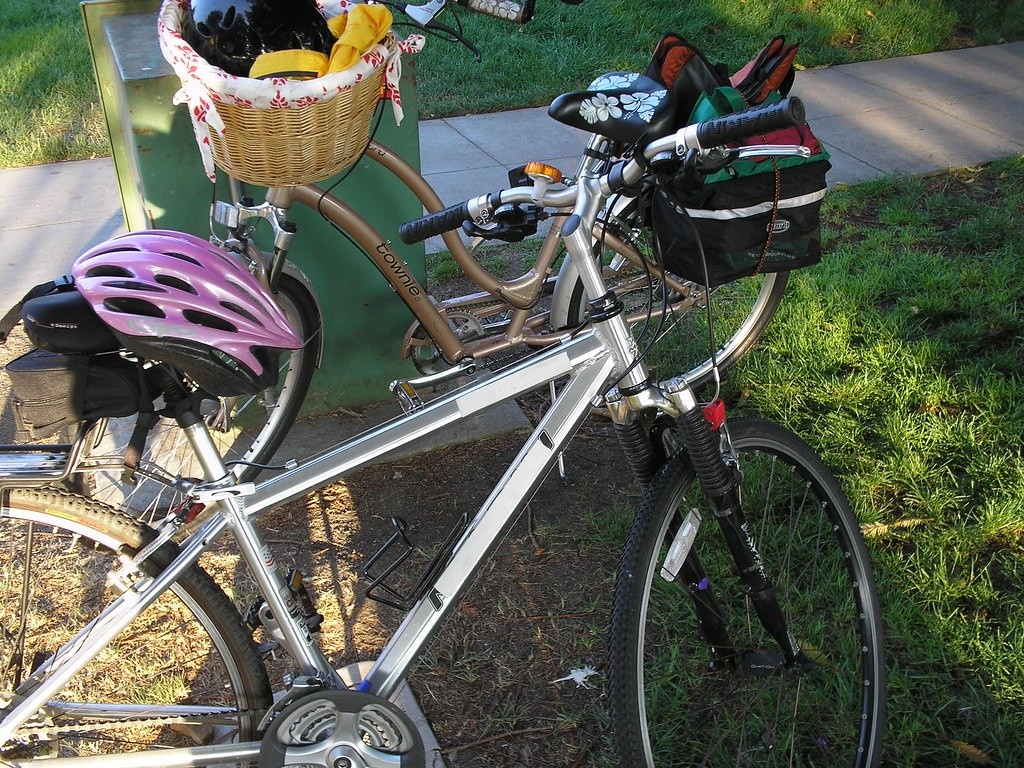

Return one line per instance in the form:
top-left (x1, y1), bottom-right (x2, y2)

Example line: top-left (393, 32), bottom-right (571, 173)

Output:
top-left (59, 0), bottom-right (792, 554)
top-left (0, 97), bottom-right (891, 767)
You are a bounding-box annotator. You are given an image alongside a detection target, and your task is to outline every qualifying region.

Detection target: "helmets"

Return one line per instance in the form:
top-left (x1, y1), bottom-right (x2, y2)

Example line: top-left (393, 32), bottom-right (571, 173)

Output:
top-left (71, 229), bottom-right (304, 399)
top-left (182, 0), bottom-right (333, 76)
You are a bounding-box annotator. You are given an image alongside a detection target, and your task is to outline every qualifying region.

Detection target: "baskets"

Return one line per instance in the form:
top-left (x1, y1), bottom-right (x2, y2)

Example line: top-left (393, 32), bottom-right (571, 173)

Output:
top-left (156, 0), bottom-right (397, 188)
